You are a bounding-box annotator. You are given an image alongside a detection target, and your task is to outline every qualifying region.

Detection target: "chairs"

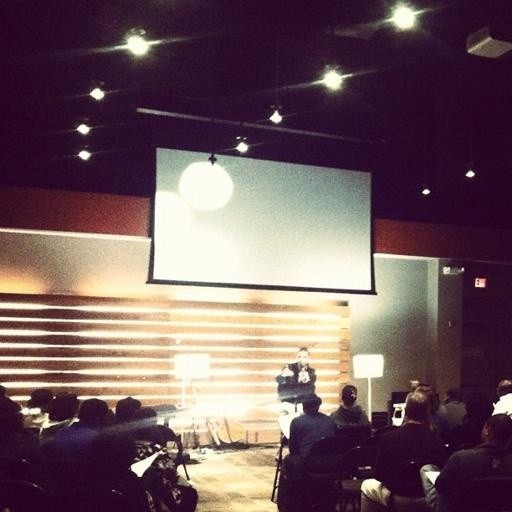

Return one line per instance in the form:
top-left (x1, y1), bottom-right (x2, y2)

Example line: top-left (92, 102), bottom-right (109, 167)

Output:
top-left (329, 426), bottom-right (512, 512)
top-left (0, 451), bottom-right (138, 512)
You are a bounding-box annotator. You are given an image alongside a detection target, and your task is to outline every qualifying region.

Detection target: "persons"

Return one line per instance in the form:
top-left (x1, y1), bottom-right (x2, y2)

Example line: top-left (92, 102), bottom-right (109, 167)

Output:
top-left (275, 347), bottom-right (317, 403)
top-left (271, 379), bottom-right (512, 512)
top-left (0, 384), bottom-right (199, 512)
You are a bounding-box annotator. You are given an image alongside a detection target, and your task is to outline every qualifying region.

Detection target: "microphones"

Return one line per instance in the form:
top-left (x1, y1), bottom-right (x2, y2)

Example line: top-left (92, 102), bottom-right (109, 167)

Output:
top-left (300, 363), bottom-right (305, 371)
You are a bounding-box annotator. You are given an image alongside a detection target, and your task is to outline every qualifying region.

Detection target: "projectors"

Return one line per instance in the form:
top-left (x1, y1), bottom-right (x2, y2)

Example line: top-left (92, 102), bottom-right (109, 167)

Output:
top-left (466, 26), bottom-right (512, 59)
